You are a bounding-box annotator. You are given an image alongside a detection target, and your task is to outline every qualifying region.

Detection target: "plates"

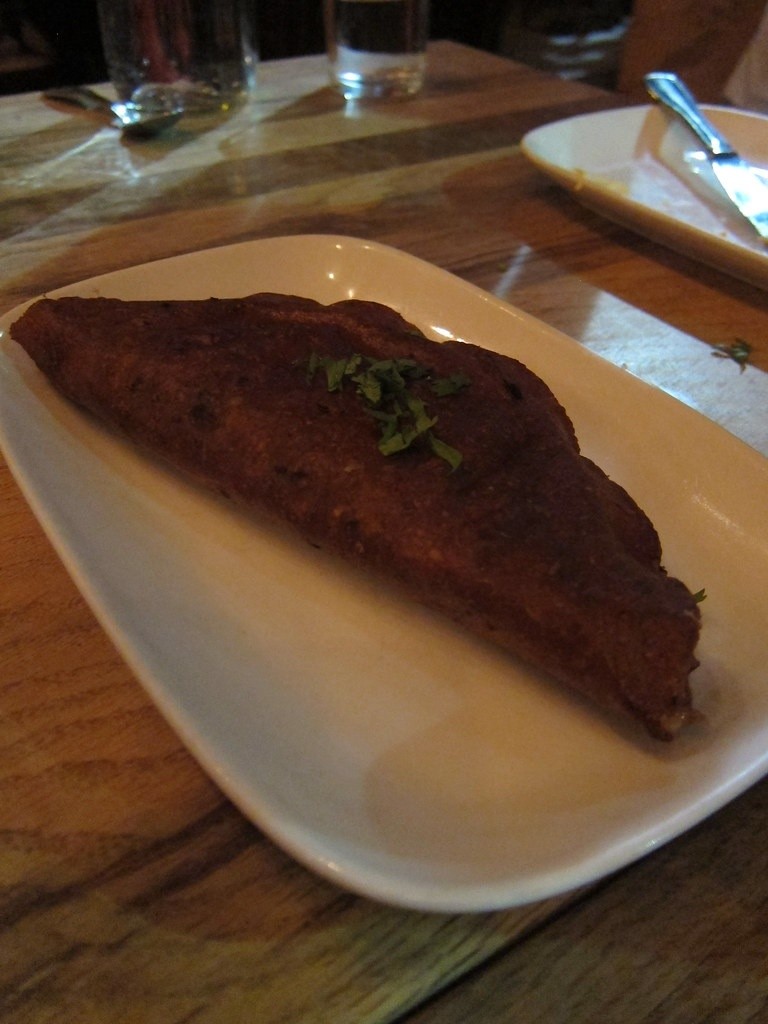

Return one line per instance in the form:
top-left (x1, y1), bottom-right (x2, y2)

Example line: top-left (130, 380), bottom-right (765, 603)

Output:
top-left (518, 100), bottom-right (767, 293)
top-left (0, 232), bottom-right (767, 917)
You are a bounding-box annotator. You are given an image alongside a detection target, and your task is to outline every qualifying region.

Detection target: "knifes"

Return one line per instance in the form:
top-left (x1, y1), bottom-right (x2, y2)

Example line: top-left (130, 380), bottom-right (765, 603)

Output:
top-left (644, 70), bottom-right (768, 244)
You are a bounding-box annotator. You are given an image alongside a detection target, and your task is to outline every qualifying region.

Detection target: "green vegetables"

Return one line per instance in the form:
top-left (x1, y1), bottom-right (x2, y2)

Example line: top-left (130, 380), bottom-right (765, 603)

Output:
top-left (306, 349), bottom-right (475, 475)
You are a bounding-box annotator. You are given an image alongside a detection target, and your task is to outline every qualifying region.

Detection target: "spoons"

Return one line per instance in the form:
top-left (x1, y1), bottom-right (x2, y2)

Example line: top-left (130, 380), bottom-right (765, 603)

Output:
top-left (46, 85), bottom-right (184, 138)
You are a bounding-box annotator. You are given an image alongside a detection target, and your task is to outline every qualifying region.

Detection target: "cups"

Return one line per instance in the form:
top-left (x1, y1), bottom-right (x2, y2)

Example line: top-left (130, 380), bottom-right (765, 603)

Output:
top-left (96, 0), bottom-right (248, 134)
top-left (324, 0), bottom-right (431, 95)
